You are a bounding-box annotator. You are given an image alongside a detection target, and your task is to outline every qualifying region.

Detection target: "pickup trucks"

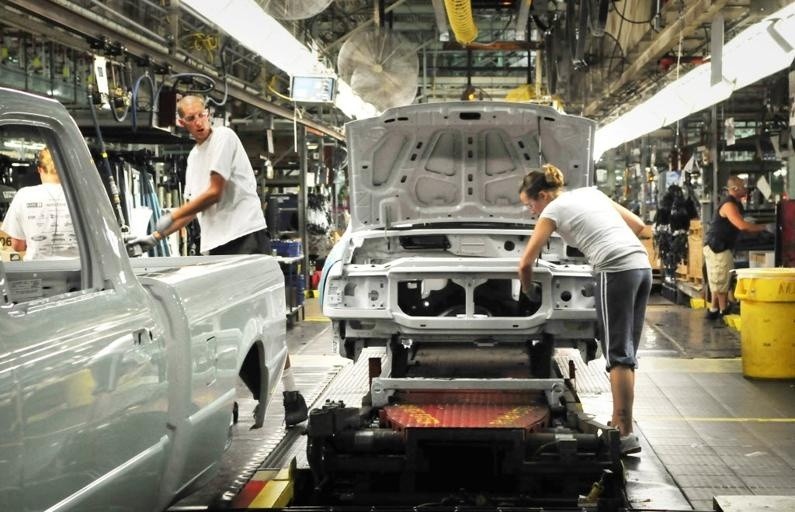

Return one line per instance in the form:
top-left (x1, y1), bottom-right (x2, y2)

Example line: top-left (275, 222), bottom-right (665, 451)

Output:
top-left (1, 85), bottom-right (287, 510)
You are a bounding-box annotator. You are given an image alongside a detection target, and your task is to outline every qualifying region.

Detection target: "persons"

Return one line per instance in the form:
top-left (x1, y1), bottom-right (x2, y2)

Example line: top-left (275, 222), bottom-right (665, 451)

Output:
top-left (122, 95), bottom-right (308, 425)
top-left (518, 163), bottom-right (653, 455)
top-left (0, 146), bottom-right (80, 261)
top-left (702, 176), bottom-right (777, 325)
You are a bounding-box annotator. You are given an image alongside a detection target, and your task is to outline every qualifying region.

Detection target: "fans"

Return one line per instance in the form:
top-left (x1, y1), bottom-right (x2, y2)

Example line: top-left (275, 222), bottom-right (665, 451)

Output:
top-left (332, 23), bottom-right (420, 110)
top-left (552, 27), bottom-right (626, 102)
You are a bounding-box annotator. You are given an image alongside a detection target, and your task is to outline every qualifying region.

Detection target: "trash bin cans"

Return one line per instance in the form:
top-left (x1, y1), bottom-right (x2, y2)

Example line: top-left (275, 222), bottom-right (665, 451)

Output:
top-left (734, 268), bottom-right (795, 379)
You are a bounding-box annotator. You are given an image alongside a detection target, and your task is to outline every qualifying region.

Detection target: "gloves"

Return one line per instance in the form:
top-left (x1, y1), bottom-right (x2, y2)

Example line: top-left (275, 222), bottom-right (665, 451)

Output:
top-left (522, 281), bottom-right (541, 303)
top-left (156, 213), bottom-right (174, 240)
top-left (765, 223), bottom-right (776, 234)
top-left (123, 234), bottom-right (159, 253)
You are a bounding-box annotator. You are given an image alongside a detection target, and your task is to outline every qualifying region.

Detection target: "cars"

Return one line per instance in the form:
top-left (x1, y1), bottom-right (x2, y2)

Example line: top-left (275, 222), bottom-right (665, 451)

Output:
top-left (319, 100), bottom-right (603, 367)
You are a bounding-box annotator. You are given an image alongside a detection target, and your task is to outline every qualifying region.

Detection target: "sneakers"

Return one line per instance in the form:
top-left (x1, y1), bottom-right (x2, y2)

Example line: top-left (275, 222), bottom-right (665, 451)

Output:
top-left (282, 390), bottom-right (308, 425)
top-left (703, 308), bottom-right (729, 329)
top-left (620, 433), bottom-right (641, 456)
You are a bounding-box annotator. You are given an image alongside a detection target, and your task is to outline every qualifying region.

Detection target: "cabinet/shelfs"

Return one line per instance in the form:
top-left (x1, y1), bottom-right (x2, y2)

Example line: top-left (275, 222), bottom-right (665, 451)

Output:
top-left (271, 254), bottom-right (306, 323)
